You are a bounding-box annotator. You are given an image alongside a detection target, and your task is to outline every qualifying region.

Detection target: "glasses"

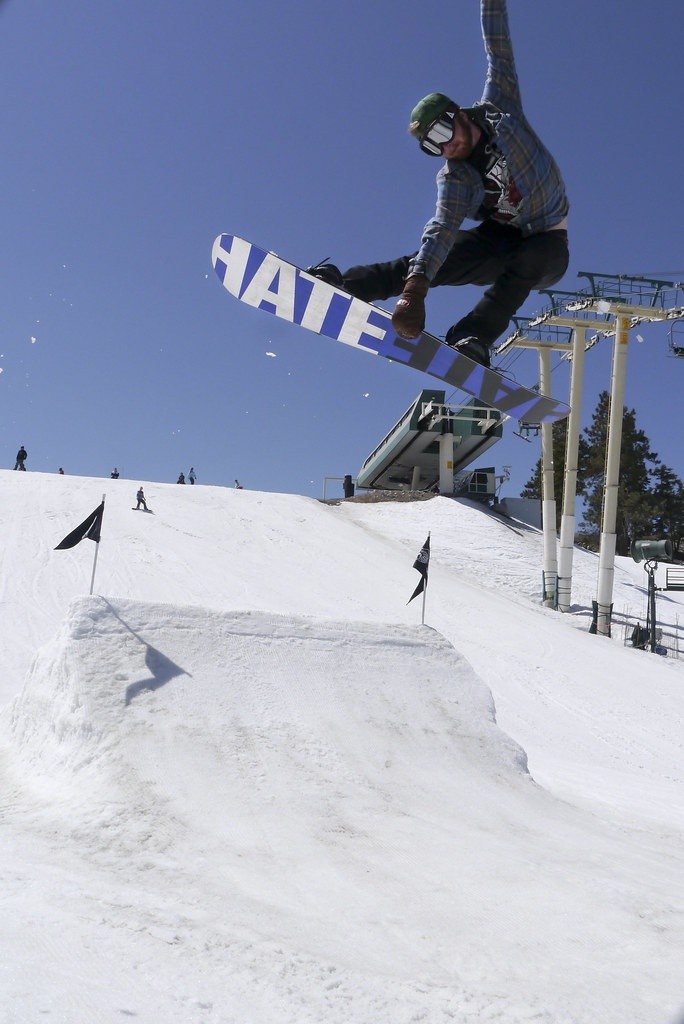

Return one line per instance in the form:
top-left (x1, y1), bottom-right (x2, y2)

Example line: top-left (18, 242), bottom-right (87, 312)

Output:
top-left (420, 113), bottom-right (455, 158)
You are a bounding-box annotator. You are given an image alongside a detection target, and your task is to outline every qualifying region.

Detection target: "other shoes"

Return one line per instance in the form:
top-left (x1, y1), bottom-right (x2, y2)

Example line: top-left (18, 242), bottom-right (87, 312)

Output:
top-left (454, 337), bottom-right (491, 369)
top-left (314, 262), bottom-right (345, 287)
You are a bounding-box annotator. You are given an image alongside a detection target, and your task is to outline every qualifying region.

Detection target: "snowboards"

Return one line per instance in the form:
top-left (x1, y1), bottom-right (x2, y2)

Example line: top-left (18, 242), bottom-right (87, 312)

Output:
top-left (132, 508), bottom-right (153, 513)
top-left (209, 232), bottom-right (572, 426)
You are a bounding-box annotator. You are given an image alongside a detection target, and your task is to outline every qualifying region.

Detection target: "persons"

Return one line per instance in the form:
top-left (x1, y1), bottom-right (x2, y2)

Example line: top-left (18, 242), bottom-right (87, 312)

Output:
top-left (179, 472), bottom-right (185, 484)
top-left (111, 468), bottom-right (119, 479)
top-left (307, 0), bottom-right (570, 368)
top-left (189, 467), bottom-right (196, 484)
top-left (235, 480), bottom-right (239, 488)
top-left (12, 446), bottom-right (27, 471)
top-left (59, 468), bottom-right (64, 474)
top-left (136, 487), bottom-right (147, 509)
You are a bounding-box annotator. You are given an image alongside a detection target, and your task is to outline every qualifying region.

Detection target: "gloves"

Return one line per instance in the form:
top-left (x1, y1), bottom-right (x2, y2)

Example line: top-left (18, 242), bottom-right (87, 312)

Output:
top-left (391, 276), bottom-right (429, 340)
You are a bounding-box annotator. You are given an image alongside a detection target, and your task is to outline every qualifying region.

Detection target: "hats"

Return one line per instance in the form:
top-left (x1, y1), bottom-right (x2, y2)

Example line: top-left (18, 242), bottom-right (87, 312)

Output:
top-left (410, 92), bottom-right (453, 140)
